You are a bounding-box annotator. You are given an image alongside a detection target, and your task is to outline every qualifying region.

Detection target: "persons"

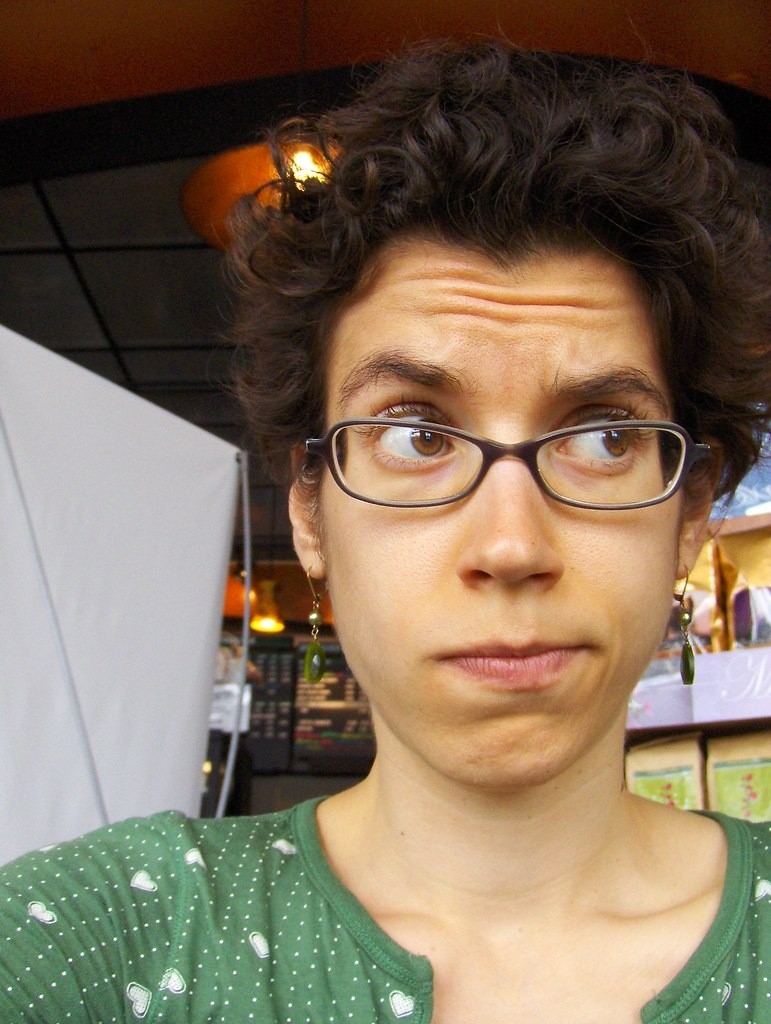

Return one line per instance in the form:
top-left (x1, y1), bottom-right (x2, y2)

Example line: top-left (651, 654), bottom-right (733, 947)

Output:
top-left (200, 640), bottom-right (262, 817)
top-left (0, 26), bottom-right (771, 1024)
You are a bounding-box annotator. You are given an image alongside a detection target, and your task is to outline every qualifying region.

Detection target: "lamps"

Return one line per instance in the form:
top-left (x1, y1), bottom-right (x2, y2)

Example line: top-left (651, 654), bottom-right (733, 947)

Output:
top-left (249, 580), bottom-right (286, 632)
top-left (182, 141), bottom-right (345, 259)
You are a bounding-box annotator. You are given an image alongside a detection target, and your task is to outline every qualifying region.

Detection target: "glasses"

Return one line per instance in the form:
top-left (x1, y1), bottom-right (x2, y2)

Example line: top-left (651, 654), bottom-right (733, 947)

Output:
top-left (301, 419), bottom-right (711, 510)
